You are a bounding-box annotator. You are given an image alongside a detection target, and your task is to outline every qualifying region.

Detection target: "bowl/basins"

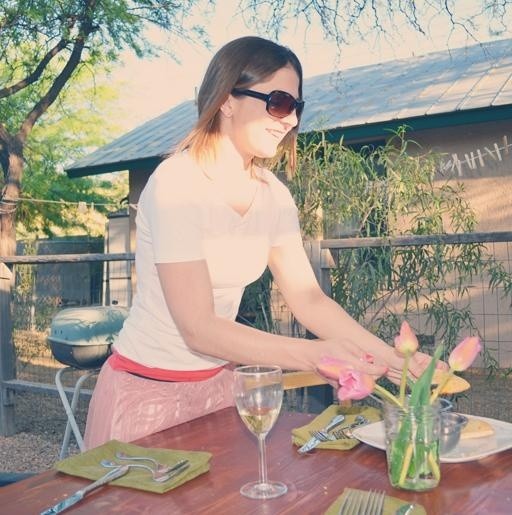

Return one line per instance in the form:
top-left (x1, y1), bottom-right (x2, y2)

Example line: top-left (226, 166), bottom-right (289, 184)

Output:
top-left (401, 393), bottom-right (469, 451)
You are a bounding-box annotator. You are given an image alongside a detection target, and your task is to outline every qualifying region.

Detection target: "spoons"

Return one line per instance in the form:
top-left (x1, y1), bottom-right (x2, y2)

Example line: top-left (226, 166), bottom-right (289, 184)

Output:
top-left (387, 368), bottom-right (438, 392)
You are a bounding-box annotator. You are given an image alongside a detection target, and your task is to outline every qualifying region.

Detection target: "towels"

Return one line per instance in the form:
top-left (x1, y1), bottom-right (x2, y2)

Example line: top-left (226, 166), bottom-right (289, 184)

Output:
top-left (50, 435), bottom-right (214, 495)
top-left (315, 484), bottom-right (430, 515)
top-left (288, 401), bottom-right (384, 453)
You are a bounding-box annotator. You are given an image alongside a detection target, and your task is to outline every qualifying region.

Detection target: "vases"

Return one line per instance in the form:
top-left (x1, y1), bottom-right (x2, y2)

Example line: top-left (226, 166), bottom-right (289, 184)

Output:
top-left (378, 393), bottom-right (456, 492)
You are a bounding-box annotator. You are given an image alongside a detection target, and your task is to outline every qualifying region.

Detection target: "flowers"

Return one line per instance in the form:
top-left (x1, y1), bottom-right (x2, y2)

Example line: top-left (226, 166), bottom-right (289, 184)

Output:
top-left (312, 314), bottom-right (488, 492)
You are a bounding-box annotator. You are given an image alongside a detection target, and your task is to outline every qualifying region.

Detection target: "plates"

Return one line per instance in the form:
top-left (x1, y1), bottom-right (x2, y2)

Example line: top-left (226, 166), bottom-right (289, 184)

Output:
top-left (349, 408), bottom-right (512, 466)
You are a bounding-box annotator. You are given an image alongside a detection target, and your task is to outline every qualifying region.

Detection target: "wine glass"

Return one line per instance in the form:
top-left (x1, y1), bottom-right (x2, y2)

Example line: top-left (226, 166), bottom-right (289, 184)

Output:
top-left (231, 363), bottom-right (288, 501)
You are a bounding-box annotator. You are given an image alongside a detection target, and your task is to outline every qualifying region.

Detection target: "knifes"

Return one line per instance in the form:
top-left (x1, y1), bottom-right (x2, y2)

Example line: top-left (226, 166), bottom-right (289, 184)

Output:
top-left (37, 463), bottom-right (129, 515)
top-left (296, 413), bottom-right (345, 456)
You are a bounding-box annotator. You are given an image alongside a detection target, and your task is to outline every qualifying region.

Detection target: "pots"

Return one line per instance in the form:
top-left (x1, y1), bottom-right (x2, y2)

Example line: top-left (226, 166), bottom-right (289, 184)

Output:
top-left (46, 305), bottom-right (131, 371)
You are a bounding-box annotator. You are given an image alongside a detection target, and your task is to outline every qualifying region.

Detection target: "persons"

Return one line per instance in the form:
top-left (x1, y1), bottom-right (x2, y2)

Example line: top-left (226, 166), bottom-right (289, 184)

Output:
top-left (84, 34), bottom-right (451, 455)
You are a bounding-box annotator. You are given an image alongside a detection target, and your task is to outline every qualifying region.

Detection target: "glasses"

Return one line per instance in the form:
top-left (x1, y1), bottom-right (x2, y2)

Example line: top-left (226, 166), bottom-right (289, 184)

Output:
top-left (230, 87), bottom-right (305, 121)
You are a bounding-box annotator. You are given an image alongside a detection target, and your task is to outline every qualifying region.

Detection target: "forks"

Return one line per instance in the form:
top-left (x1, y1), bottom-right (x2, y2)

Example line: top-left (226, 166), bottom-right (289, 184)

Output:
top-left (305, 416), bottom-right (367, 441)
top-left (336, 489), bottom-right (389, 515)
top-left (101, 451), bottom-right (190, 484)
